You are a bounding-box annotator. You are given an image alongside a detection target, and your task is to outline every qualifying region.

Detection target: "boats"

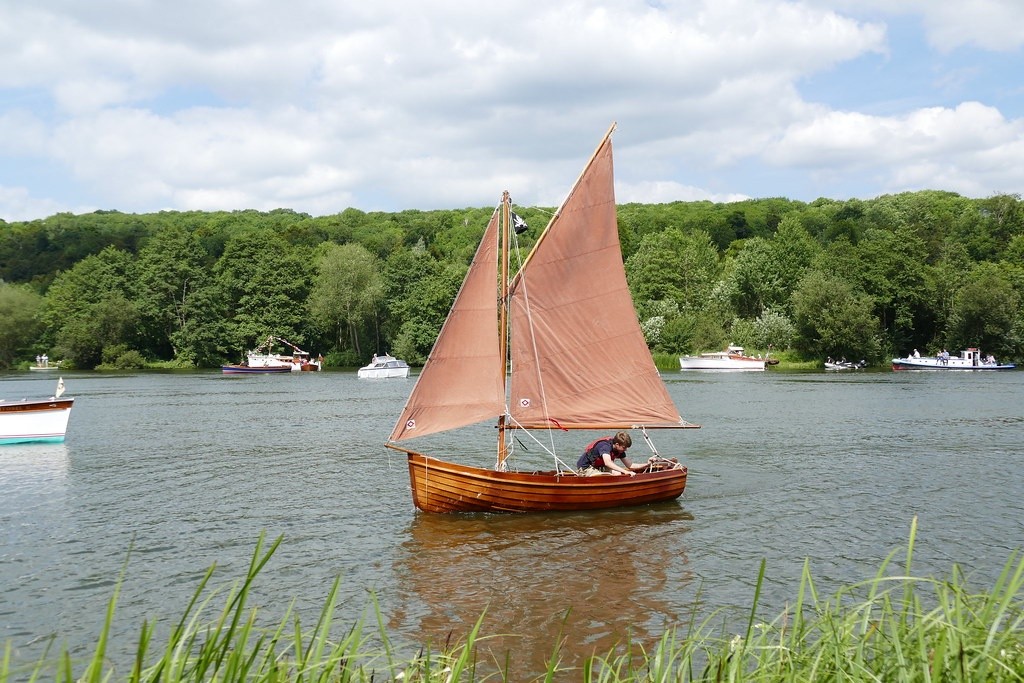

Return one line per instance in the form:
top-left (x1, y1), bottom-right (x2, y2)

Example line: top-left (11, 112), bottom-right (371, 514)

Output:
top-left (221, 335), bottom-right (319, 374)
top-left (678, 342), bottom-right (780, 371)
top-left (890, 347), bottom-right (1016, 371)
top-left (0, 377), bottom-right (77, 446)
top-left (824, 360), bottom-right (869, 371)
top-left (357, 351), bottom-right (412, 378)
top-left (28, 364), bottom-right (59, 371)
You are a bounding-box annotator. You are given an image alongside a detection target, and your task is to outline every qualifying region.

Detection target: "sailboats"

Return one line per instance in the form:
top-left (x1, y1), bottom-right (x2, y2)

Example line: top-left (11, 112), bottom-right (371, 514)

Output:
top-left (380, 121), bottom-right (704, 515)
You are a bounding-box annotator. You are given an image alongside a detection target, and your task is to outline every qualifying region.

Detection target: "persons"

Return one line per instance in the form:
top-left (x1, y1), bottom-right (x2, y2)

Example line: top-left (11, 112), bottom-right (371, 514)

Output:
top-left (576, 430), bottom-right (657, 478)
top-left (728, 350), bottom-right (762, 359)
top-left (827, 354), bottom-right (868, 371)
top-left (936, 348), bottom-right (950, 366)
top-left (912, 349), bottom-right (920, 358)
